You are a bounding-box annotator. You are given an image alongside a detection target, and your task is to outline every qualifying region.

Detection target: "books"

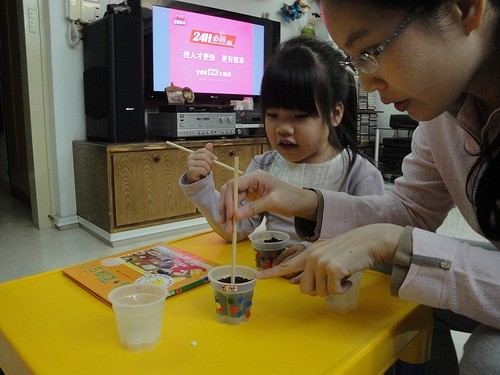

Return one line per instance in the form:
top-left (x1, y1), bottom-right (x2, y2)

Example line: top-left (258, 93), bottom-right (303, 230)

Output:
top-left (63, 241), bottom-right (222, 308)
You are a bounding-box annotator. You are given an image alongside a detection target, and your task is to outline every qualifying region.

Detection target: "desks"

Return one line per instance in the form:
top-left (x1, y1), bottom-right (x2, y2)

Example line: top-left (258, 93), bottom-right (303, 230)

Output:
top-left (0, 224), bottom-right (436, 375)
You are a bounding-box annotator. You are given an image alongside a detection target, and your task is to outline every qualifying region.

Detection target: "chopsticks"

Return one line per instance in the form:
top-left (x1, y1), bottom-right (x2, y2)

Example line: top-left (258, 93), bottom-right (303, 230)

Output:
top-left (165, 141), bottom-right (243, 284)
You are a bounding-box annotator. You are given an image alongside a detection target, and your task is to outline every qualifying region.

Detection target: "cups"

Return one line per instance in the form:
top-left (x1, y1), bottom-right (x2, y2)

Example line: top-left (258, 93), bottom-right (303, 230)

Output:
top-left (243, 97), bottom-right (254, 110)
top-left (108, 282), bottom-right (168, 353)
top-left (207, 265), bottom-right (260, 326)
top-left (247, 229), bottom-right (291, 271)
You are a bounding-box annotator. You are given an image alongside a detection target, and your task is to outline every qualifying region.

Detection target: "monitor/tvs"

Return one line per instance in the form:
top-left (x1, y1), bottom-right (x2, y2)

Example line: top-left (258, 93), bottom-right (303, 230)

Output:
top-left (137, 0), bottom-right (280, 111)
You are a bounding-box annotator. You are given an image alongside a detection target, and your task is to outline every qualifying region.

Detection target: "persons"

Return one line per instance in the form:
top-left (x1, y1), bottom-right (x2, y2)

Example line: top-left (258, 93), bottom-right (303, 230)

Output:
top-left (179, 36), bottom-right (383, 283)
top-left (218, 0), bottom-right (500, 375)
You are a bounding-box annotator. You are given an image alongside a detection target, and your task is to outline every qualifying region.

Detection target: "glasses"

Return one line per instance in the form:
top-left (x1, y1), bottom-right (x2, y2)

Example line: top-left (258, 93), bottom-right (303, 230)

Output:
top-left (337, 0), bottom-right (435, 75)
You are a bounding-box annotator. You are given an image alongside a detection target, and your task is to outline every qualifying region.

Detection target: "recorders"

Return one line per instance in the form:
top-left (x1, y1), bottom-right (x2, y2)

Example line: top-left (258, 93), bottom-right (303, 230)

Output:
top-left (83, 13), bottom-right (146, 144)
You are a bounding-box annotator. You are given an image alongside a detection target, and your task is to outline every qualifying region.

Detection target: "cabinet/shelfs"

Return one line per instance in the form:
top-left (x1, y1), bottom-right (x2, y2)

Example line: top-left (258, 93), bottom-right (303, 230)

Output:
top-left (71, 137), bottom-right (269, 248)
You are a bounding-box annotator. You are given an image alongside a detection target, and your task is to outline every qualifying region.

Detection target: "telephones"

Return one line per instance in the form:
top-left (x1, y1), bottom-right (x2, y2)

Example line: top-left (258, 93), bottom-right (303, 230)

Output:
top-left (64, 0), bottom-right (103, 24)
